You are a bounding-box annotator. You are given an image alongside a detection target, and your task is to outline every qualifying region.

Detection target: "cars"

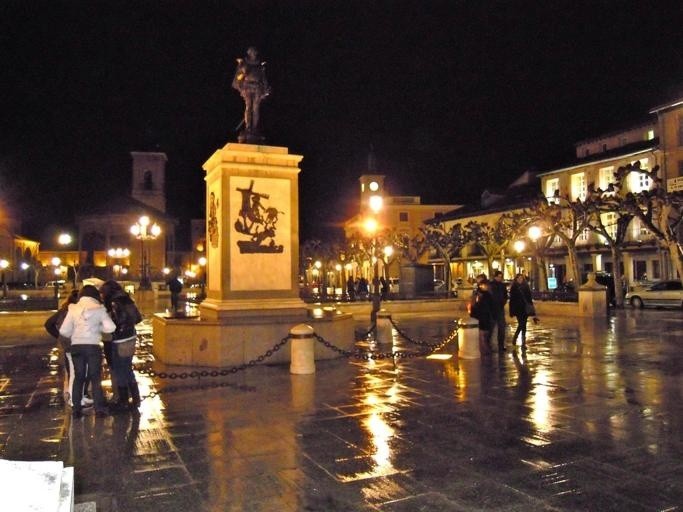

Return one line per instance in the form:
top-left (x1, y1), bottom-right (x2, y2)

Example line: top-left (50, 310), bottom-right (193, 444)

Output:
top-left (3, 280), bottom-right (36, 288)
top-left (625, 278), bottom-right (683, 310)
top-left (383, 275), bottom-right (458, 297)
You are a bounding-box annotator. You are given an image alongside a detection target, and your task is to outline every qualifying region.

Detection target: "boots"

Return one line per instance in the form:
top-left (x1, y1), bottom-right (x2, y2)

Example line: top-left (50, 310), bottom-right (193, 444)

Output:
top-left (109, 382), bottom-right (140, 411)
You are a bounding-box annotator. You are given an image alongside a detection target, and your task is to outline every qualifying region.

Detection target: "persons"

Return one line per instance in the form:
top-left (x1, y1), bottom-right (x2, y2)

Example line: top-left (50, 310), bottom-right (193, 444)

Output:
top-left (482, 269), bottom-right (510, 353)
top-left (346, 275), bottom-right (388, 301)
top-left (468, 272), bottom-right (494, 353)
top-left (232, 45), bottom-right (269, 132)
top-left (40, 279), bottom-right (143, 419)
top-left (508, 272), bottom-right (536, 349)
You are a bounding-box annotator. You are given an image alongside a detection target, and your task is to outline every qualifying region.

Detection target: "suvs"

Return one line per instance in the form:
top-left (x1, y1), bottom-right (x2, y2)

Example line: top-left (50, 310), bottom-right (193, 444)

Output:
top-left (550, 271), bottom-right (628, 304)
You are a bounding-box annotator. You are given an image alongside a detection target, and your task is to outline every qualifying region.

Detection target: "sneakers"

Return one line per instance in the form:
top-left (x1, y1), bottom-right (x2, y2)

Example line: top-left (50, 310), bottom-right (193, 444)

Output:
top-left (67, 396), bottom-right (107, 419)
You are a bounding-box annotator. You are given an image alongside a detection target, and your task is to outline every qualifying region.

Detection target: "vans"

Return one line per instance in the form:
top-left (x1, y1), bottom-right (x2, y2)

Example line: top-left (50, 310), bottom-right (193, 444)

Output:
top-left (44, 279), bottom-right (65, 290)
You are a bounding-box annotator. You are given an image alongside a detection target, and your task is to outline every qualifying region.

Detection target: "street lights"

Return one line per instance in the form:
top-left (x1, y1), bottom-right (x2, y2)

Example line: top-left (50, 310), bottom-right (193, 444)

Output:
top-left (108, 246), bottom-right (131, 282)
top-left (513, 225), bottom-right (541, 297)
top-left (130, 215), bottom-right (162, 290)
top-left (358, 191), bottom-right (394, 344)
